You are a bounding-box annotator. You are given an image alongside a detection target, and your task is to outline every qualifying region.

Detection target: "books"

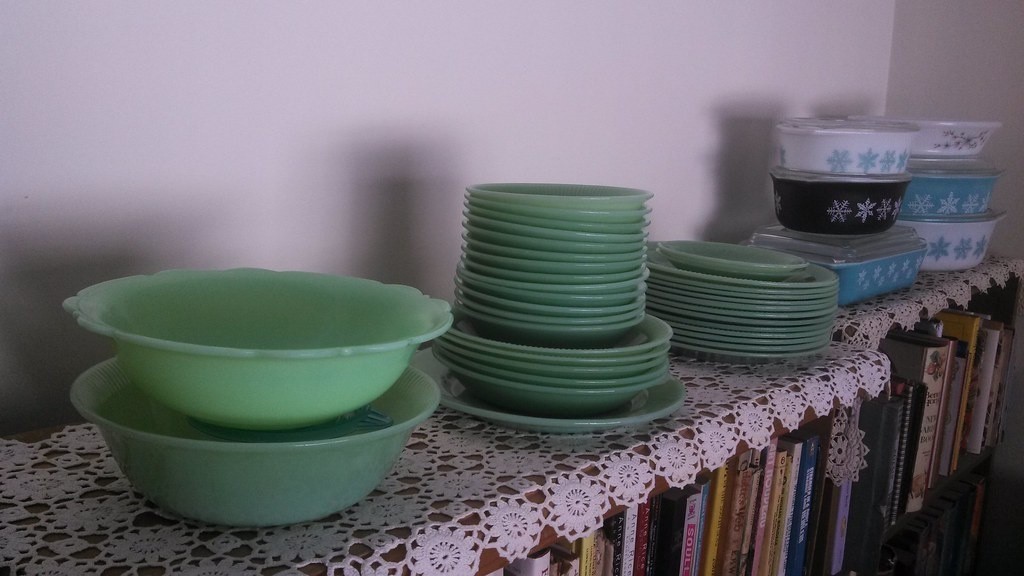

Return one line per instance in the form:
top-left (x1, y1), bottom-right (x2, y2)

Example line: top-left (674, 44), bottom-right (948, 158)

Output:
top-left (468, 288), bottom-right (1017, 576)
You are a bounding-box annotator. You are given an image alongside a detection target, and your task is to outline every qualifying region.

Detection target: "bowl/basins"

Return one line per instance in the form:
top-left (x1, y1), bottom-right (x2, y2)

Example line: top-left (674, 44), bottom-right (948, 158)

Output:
top-left (769, 119), bottom-right (1010, 307)
top-left (60, 267), bottom-right (454, 527)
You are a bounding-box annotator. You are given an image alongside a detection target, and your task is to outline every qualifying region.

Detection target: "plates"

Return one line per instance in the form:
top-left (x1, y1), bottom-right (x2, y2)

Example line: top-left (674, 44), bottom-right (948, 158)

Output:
top-left (430, 184), bottom-right (687, 433)
top-left (646, 240), bottom-right (839, 364)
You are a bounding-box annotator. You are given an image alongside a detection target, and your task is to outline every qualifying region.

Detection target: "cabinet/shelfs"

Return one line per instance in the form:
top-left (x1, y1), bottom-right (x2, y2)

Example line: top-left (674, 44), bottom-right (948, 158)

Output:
top-left (830, 254), bottom-right (1024, 576)
top-left (0, 341), bottom-right (892, 576)
